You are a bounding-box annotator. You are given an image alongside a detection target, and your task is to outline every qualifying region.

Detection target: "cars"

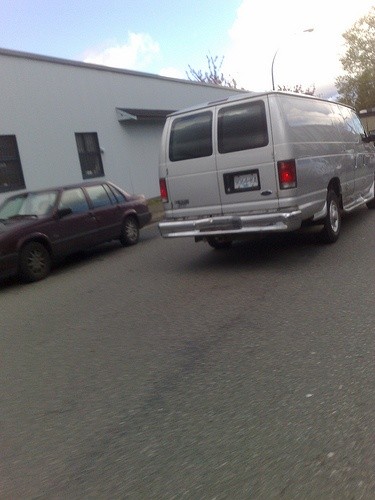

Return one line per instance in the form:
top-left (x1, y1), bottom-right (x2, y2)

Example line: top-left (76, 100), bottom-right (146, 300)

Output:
top-left (0, 176), bottom-right (153, 285)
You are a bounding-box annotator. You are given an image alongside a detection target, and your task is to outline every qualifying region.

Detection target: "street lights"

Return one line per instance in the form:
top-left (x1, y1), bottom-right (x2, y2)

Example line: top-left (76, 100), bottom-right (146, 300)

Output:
top-left (271, 27), bottom-right (317, 90)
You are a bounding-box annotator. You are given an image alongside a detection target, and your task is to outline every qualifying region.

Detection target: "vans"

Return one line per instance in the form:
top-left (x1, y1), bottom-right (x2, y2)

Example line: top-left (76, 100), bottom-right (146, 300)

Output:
top-left (156, 88), bottom-right (375, 251)
